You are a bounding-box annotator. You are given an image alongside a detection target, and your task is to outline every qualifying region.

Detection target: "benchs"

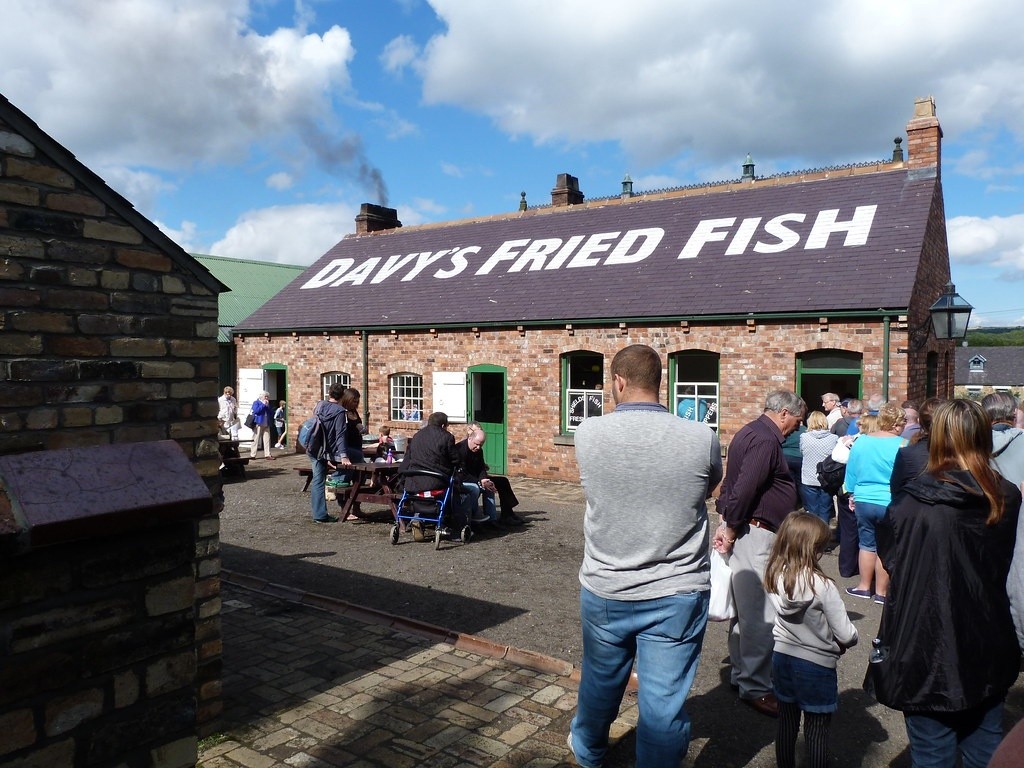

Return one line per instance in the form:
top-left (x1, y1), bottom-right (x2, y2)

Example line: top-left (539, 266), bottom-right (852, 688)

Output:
top-left (222, 457), bottom-right (256, 462)
top-left (293, 468), bottom-right (411, 522)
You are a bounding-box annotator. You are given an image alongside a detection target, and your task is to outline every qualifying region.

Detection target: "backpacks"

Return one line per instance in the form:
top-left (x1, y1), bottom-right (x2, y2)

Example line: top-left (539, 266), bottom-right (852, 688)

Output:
top-left (297, 399), bottom-right (328, 462)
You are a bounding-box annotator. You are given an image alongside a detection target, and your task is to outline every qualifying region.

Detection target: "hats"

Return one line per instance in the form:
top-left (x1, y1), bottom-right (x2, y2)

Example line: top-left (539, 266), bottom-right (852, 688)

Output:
top-left (838, 398), bottom-right (852, 408)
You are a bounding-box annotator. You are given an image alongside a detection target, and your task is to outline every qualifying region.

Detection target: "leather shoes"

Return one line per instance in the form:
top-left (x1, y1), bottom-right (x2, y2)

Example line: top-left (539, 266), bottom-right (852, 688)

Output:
top-left (731, 682), bottom-right (739, 690)
top-left (751, 694), bottom-right (778, 718)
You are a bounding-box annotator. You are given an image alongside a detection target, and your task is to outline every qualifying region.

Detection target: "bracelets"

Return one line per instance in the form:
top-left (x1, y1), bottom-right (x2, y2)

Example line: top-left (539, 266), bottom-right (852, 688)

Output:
top-left (722, 523), bottom-right (734, 543)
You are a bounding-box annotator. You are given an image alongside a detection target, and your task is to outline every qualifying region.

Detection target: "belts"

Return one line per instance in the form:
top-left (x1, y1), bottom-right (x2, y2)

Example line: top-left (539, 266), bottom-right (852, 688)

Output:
top-left (749, 519), bottom-right (774, 533)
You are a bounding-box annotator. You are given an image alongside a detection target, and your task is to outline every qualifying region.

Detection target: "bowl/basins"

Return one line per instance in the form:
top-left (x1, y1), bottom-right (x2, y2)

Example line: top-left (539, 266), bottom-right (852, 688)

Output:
top-left (363, 458), bottom-right (372, 463)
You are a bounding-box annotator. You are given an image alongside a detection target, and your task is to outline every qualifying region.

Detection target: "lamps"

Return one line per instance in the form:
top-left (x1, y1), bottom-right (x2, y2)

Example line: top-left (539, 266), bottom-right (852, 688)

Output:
top-left (896, 279), bottom-right (975, 354)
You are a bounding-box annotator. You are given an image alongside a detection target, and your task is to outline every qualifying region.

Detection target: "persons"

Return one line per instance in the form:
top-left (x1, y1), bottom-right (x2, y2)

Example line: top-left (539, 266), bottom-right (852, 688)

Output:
top-left (782, 393), bottom-right (1024, 658)
top-left (567, 344), bottom-right (723, 768)
top-left (401, 399), bottom-right (420, 421)
top-left (677, 385), bottom-right (707, 422)
top-left (372, 426), bottom-right (398, 494)
top-left (712, 388), bottom-right (808, 718)
top-left (338, 388), bottom-right (364, 520)
top-left (588, 384), bottom-right (602, 417)
top-left (862, 398), bottom-right (1024, 768)
top-left (274, 400), bottom-right (286, 449)
top-left (250, 392), bottom-right (275, 460)
top-left (304, 383), bottom-right (352, 523)
top-left (763, 511), bottom-right (858, 768)
top-left (218, 386), bottom-right (239, 441)
top-left (397, 412), bottom-right (524, 541)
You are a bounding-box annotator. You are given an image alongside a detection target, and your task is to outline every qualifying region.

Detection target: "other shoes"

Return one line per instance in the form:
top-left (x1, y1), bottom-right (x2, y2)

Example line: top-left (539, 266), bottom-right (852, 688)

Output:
top-left (275, 443), bottom-right (284, 449)
top-left (847, 586), bottom-right (870, 598)
top-left (265, 455), bottom-right (275, 460)
top-left (875, 594), bottom-right (885, 605)
top-left (346, 514), bottom-right (358, 520)
top-left (473, 512), bottom-right (491, 522)
top-left (500, 514), bottom-right (523, 525)
top-left (314, 513), bottom-right (337, 523)
top-left (352, 510), bottom-right (367, 517)
top-left (566, 730), bottom-right (575, 754)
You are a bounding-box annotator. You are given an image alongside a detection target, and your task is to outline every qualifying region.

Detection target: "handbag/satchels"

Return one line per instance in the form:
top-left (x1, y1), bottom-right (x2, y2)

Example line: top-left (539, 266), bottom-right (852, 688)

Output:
top-left (706, 546), bottom-right (738, 622)
top-left (244, 414), bottom-right (256, 429)
top-left (817, 460), bottom-right (846, 491)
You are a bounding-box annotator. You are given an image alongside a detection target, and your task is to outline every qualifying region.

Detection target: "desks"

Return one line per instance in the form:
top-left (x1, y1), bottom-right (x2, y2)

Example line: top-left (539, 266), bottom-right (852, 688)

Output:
top-left (337, 463), bottom-right (406, 532)
top-left (326, 447), bottom-right (404, 487)
top-left (218, 439), bottom-right (254, 482)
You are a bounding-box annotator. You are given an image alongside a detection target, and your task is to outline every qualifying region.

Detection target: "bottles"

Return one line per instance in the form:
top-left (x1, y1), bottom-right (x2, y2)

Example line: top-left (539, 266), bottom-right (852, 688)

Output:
top-left (869, 639), bottom-right (887, 664)
top-left (388, 449), bottom-right (392, 464)
top-left (362, 434), bottom-right (379, 440)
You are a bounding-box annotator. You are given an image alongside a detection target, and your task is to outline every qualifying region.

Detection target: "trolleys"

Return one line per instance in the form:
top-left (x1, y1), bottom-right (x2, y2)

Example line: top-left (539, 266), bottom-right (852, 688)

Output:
top-left (388, 467), bottom-right (475, 550)
